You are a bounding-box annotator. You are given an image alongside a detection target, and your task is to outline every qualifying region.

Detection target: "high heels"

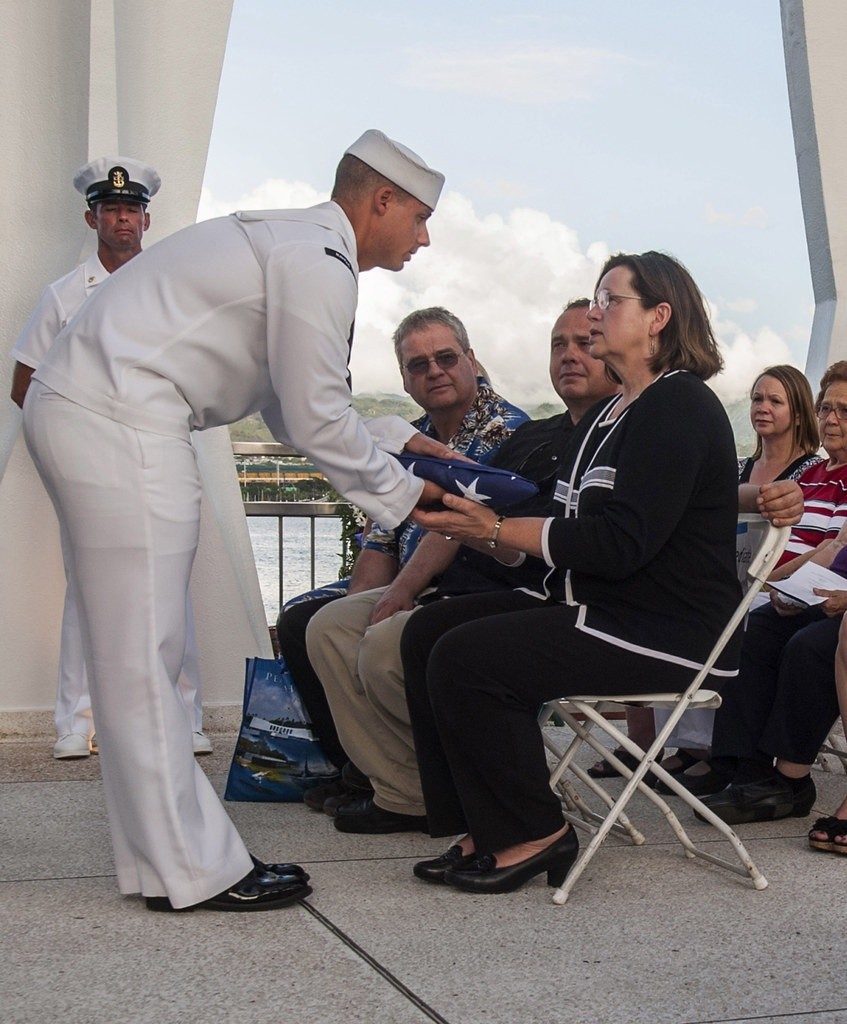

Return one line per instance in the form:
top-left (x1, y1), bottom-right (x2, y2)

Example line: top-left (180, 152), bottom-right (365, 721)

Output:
top-left (413, 819), bottom-right (580, 894)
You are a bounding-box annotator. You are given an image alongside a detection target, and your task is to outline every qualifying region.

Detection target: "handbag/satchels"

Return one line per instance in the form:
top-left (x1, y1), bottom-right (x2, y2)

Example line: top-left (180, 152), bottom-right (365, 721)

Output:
top-left (224, 657), bottom-right (340, 802)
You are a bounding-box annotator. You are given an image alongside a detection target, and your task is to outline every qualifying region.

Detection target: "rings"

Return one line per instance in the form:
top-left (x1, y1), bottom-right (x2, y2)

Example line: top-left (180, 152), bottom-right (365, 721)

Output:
top-left (445, 536), bottom-right (454, 541)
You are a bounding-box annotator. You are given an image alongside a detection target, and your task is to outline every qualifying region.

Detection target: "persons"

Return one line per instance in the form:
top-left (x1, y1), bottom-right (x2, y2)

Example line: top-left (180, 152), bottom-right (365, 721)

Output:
top-left (305, 300), bottom-right (804, 835)
top-left (401, 251), bottom-right (743, 897)
top-left (585, 362), bottom-right (847, 853)
top-left (277, 310), bottom-right (530, 816)
top-left (24, 129), bottom-right (479, 913)
top-left (8, 157), bottom-right (210, 758)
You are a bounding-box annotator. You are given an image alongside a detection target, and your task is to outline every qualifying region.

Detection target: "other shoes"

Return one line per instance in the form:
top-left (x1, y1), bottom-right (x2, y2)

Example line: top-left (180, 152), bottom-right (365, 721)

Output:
top-left (193, 732), bottom-right (213, 752)
top-left (304, 778), bottom-right (429, 833)
top-left (587, 748), bottom-right (664, 778)
top-left (693, 762), bottom-right (817, 825)
top-left (54, 732), bottom-right (90, 758)
top-left (642, 748), bottom-right (764, 796)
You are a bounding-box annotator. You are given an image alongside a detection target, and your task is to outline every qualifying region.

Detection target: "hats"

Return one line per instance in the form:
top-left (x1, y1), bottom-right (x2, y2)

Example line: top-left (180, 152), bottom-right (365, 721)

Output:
top-left (344, 129), bottom-right (445, 212)
top-left (73, 155), bottom-right (161, 206)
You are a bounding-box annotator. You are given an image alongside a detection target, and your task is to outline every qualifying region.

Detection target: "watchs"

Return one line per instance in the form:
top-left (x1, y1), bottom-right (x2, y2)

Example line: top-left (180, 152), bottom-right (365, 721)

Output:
top-left (487, 515), bottom-right (505, 549)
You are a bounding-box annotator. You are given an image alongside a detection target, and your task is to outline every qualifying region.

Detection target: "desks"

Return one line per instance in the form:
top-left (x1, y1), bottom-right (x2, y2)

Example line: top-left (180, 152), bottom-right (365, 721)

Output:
top-left (243, 500), bottom-right (353, 623)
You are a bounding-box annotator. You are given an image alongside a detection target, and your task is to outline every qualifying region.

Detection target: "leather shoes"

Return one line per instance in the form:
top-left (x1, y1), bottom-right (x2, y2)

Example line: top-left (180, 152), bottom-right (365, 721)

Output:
top-left (146, 853), bottom-right (313, 913)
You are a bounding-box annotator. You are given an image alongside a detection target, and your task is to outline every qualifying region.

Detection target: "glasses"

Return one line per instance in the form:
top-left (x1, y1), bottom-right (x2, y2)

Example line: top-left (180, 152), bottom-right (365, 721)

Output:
top-left (589, 288), bottom-right (648, 311)
top-left (818, 404), bottom-right (847, 420)
top-left (401, 352), bottom-right (464, 376)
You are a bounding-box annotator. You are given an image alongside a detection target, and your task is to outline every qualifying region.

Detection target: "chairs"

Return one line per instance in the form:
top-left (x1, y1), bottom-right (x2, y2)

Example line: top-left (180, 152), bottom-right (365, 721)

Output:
top-left (547, 507), bottom-right (847, 906)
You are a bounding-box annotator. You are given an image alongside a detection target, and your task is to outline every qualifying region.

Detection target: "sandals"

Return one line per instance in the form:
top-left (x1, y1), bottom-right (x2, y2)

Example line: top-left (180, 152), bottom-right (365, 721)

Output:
top-left (808, 816), bottom-right (847, 853)
